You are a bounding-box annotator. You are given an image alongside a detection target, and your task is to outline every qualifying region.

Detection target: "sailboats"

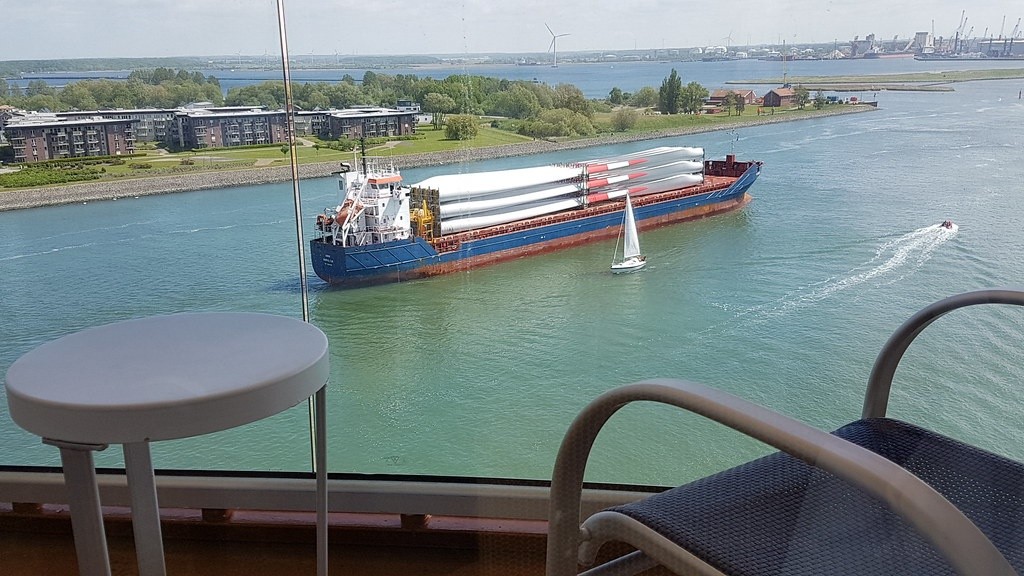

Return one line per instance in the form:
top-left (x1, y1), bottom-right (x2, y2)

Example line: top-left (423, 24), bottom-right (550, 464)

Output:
top-left (609, 189), bottom-right (648, 274)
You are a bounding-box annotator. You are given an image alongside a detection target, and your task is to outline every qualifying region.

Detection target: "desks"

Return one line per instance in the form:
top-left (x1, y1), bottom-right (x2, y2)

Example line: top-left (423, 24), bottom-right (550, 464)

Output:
top-left (4, 310), bottom-right (331, 576)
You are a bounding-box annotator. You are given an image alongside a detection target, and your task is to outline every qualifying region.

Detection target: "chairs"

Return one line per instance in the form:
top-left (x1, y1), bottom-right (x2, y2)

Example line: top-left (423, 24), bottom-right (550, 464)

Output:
top-left (546, 290), bottom-right (1024, 576)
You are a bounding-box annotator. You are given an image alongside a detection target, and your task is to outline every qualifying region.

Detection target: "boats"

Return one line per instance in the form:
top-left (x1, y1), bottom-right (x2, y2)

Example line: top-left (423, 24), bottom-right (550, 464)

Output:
top-left (308, 135), bottom-right (764, 287)
